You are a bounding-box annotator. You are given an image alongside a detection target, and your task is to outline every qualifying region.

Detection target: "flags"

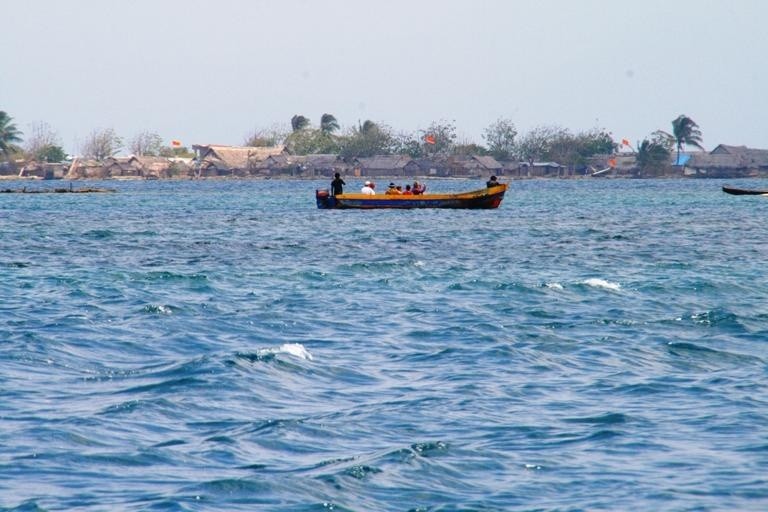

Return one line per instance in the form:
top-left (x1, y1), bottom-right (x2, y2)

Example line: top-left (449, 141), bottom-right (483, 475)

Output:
top-left (171, 141), bottom-right (181, 146)
top-left (425, 136), bottom-right (436, 145)
top-left (620, 139), bottom-right (628, 145)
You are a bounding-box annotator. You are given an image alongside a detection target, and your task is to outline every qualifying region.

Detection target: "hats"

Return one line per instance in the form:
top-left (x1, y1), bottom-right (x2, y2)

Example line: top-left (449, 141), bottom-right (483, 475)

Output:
top-left (364, 180), bottom-right (420, 188)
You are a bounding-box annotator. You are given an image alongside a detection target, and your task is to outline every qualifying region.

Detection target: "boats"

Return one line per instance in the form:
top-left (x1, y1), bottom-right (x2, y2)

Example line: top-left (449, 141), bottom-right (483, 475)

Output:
top-left (723, 180), bottom-right (768, 198)
top-left (316, 181), bottom-right (509, 211)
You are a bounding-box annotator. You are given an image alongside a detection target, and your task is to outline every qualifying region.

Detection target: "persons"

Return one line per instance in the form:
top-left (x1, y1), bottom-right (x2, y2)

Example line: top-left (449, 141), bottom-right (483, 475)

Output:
top-left (401, 184), bottom-right (413, 195)
top-left (484, 174), bottom-right (499, 189)
top-left (409, 180), bottom-right (427, 195)
top-left (383, 182), bottom-right (401, 195)
top-left (329, 172), bottom-right (346, 197)
top-left (359, 180), bottom-right (375, 196)
top-left (396, 185), bottom-right (402, 192)
